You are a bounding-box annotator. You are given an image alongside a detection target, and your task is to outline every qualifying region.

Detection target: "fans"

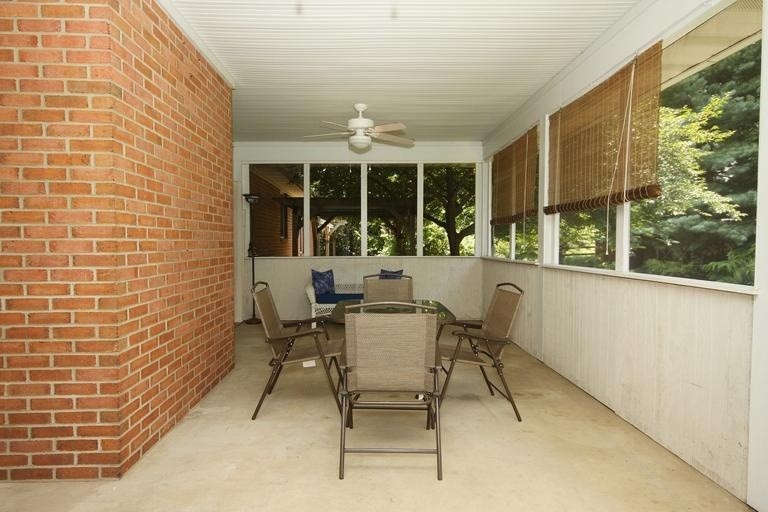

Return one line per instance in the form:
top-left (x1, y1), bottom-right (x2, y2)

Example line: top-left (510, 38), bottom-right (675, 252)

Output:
top-left (303, 103), bottom-right (415, 148)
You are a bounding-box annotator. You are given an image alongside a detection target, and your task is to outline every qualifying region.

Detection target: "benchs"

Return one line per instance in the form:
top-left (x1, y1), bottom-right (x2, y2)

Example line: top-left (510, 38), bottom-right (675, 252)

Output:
top-left (306, 283), bottom-right (363, 335)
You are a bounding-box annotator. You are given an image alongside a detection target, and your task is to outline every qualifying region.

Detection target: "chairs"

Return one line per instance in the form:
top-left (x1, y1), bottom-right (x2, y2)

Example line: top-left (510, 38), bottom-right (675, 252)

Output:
top-left (362, 274), bottom-right (414, 303)
top-left (251, 282), bottom-right (344, 420)
top-left (433, 282), bottom-right (525, 422)
top-left (338, 302), bottom-right (442, 480)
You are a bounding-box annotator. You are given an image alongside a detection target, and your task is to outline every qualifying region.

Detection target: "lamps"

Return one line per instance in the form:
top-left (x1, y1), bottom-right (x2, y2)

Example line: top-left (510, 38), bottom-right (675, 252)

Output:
top-left (349, 136), bottom-right (371, 150)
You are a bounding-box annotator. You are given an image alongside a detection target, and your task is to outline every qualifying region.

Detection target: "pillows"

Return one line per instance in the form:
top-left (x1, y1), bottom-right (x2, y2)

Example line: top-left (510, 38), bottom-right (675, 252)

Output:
top-left (311, 268), bottom-right (334, 294)
top-left (379, 269), bottom-right (404, 279)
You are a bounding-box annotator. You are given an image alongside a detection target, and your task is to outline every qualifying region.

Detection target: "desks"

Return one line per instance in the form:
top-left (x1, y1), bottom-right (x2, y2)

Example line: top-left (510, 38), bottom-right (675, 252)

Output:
top-left (331, 300), bottom-right (456, 429)
